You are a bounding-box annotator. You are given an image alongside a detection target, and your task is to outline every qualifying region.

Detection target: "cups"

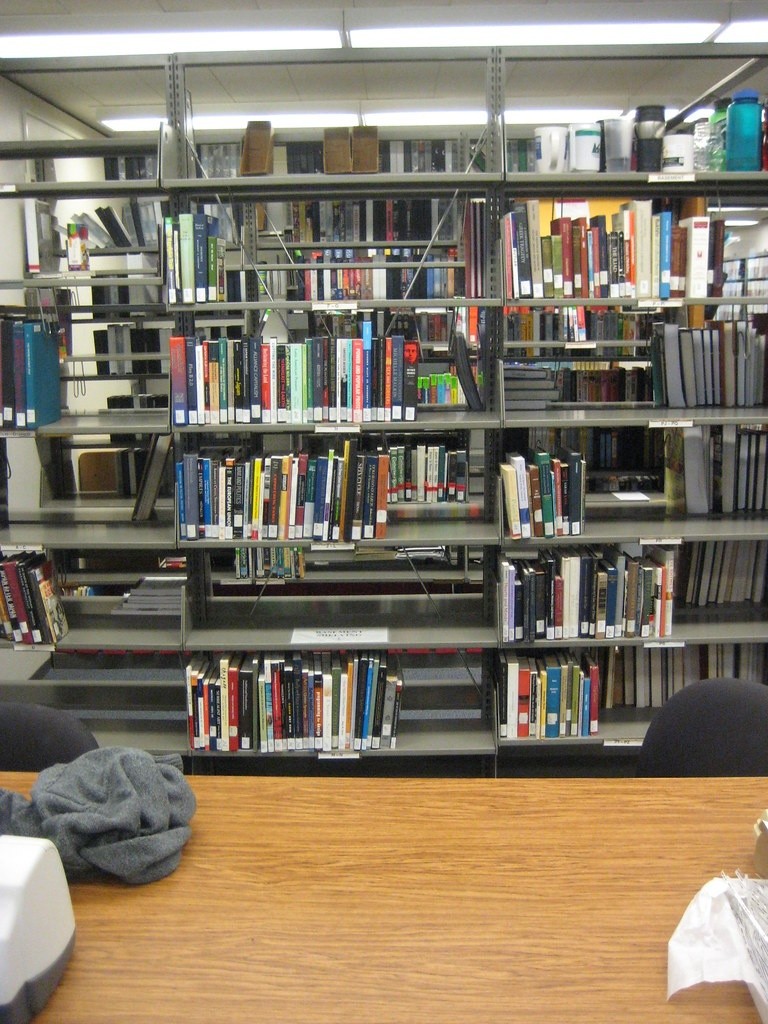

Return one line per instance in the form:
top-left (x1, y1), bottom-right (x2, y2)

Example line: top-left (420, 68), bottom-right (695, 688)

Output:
top-left (661, 134), bottom-right (693, 173)
top-left (533, 119), bottom-right (634, 173)
top-left (631, 105), bottom-right (667, 172)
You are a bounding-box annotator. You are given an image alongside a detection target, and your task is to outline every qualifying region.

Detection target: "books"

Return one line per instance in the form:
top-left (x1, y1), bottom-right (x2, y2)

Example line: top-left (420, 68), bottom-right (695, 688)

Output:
top-left (115, 449), bottom-right (147, 496)
top-left (24, 196), bottom-right (486, 318)
top-left (505, 306), bottom-right (767, 410)
top-left (177, 432), bottom-right (468, 541)
top-left (185, 651), bottom-right (404, 753)
top-left (499, 540), bottom-right (768, 642)
top-left (0, 551), bottom-right (99, 643)
top-left (499, 425), bottom-right (768, 538)
top-left (92, 310), bottom-right (480, 426)
top-left (0, 317), bottom-right (61, 429)
top-left (503, 194), bottom-right (767, 298)
top-left (110, 577), bottom-right (188, 616)
top-left (104, 141), bottom-right (533, 179)
top-left (490, 641), bottom-right (768, 738)
top-left (165, 555), bottom-right (187, 569)
top-left (235, 542), bottom-right (448, 578)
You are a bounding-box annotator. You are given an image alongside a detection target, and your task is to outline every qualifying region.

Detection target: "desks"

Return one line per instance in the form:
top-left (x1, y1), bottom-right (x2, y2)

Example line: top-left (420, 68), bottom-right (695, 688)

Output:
top-left (1, 773), bottom-right (768, 1024)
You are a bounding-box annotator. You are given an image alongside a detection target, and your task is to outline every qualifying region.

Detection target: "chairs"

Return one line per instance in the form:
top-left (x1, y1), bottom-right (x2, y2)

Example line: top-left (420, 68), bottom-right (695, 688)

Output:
top-left (638, 679), bottom-right (768, 777)
top-left (1, 699), bottom-right (99, 773)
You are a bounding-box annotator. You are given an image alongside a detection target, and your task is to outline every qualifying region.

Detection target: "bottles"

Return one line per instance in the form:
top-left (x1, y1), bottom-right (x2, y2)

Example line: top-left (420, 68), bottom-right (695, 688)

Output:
top-left (693, 88), bottom-right (768, 172)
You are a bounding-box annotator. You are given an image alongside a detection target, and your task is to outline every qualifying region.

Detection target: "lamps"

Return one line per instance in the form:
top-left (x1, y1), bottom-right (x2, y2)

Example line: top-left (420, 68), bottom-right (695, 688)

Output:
top-left (0, 1), bottom-right (768, 61)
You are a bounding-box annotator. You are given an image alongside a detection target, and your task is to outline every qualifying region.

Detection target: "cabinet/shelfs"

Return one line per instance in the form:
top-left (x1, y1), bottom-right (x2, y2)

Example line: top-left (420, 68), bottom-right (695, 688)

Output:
top-left (1, 43), bottom-right (767, 780)
